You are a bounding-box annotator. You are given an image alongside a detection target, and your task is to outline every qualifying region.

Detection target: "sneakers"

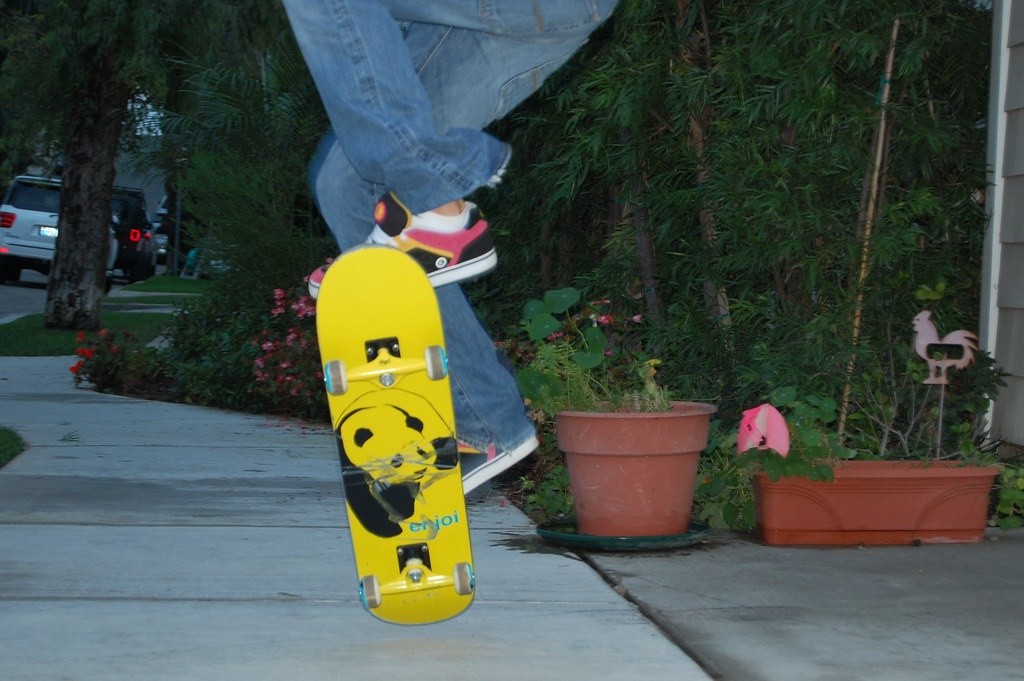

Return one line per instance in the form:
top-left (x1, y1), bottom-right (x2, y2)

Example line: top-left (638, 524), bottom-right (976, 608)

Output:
top-left (307, 193), bottom-right (499, 304)
top-left (455, 425), bottom-right (539, 498)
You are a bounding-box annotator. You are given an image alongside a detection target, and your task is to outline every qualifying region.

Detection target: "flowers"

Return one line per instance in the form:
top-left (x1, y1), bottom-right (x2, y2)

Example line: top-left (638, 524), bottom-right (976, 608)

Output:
top-left (509, 287), bottom-right (677, 414)
top-left (719, 351), bottom-right (1020, 482)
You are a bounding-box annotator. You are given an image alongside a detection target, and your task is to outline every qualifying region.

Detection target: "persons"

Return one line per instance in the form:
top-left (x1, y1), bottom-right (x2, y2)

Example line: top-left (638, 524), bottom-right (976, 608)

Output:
top-left (281, 0), bottom-right (619, 495)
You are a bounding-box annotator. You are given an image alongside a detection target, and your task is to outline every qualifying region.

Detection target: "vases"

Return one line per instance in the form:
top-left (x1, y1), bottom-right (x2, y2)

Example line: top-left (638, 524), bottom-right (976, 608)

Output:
top-left (556, 400), bottom-right (718, 533)
top-left (753, 459), bottom-right (1001, 545)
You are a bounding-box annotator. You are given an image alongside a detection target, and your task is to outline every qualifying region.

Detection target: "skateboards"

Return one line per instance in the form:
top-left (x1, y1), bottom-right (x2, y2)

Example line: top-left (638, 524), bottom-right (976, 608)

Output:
top-left (313, 244), bottom-right (481, 624)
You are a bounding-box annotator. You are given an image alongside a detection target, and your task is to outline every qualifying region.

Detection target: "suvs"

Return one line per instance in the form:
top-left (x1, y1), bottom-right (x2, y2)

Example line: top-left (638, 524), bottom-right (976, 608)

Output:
top-left (0, 177), bottom-right (169, 295)
